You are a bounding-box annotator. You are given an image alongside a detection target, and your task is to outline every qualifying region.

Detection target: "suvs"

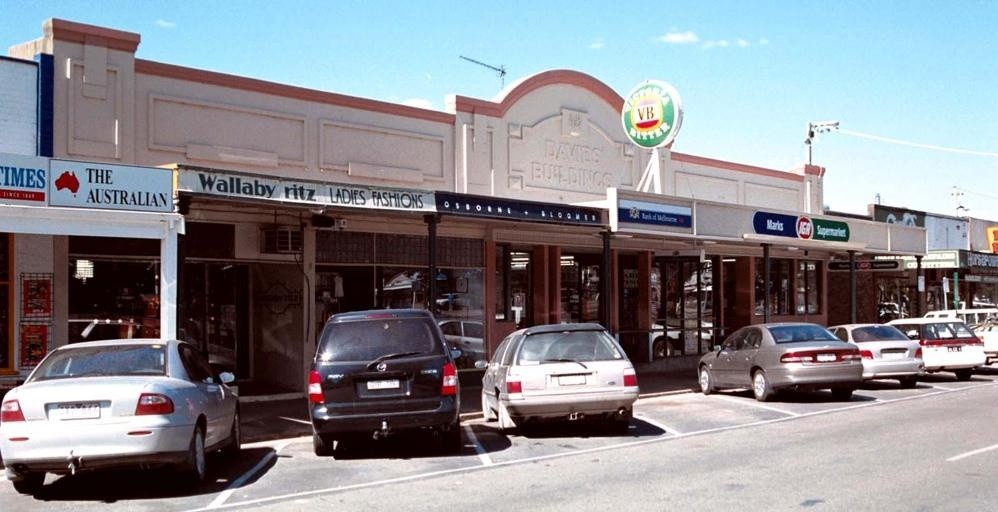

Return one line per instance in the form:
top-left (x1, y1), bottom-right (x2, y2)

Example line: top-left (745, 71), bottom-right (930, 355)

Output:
top-left (878, 303), bottom-right (909, 319)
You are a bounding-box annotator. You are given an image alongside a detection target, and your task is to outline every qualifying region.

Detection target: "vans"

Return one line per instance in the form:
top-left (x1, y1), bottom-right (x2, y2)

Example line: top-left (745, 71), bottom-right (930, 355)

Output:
top-left (307, 305), bottom-right (466, 458)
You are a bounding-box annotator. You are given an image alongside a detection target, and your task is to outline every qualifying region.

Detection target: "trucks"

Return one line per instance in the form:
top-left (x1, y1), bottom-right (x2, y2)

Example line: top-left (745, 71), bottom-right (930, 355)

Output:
top-left (923, 308), bottom-right (998, 374)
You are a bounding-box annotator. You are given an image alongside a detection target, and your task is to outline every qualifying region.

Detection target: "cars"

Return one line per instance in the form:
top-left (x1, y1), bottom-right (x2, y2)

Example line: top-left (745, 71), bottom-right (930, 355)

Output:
top-left (432, 294), bottom-right (465, 311)
top-left (1, 336), bottom-right (246, 495)
top-left (880, 317), bottom-right (986, 381)
top-left (826, 324), bottom-right (925, 390)
top-left (202, 315), bottom-right (235, 379)
top-left (695, 321), bottom-right (863, 402)
top-left (68, 318), bottom-right (151, 342)
top-left (474, 322), bottom-right (637, 436)
top-left (435, 317), bottom-right (485, 367)
top-left (650, 317), bottom-right (713, 359)
top-left (755, 303), bottom-right (819, 315)
